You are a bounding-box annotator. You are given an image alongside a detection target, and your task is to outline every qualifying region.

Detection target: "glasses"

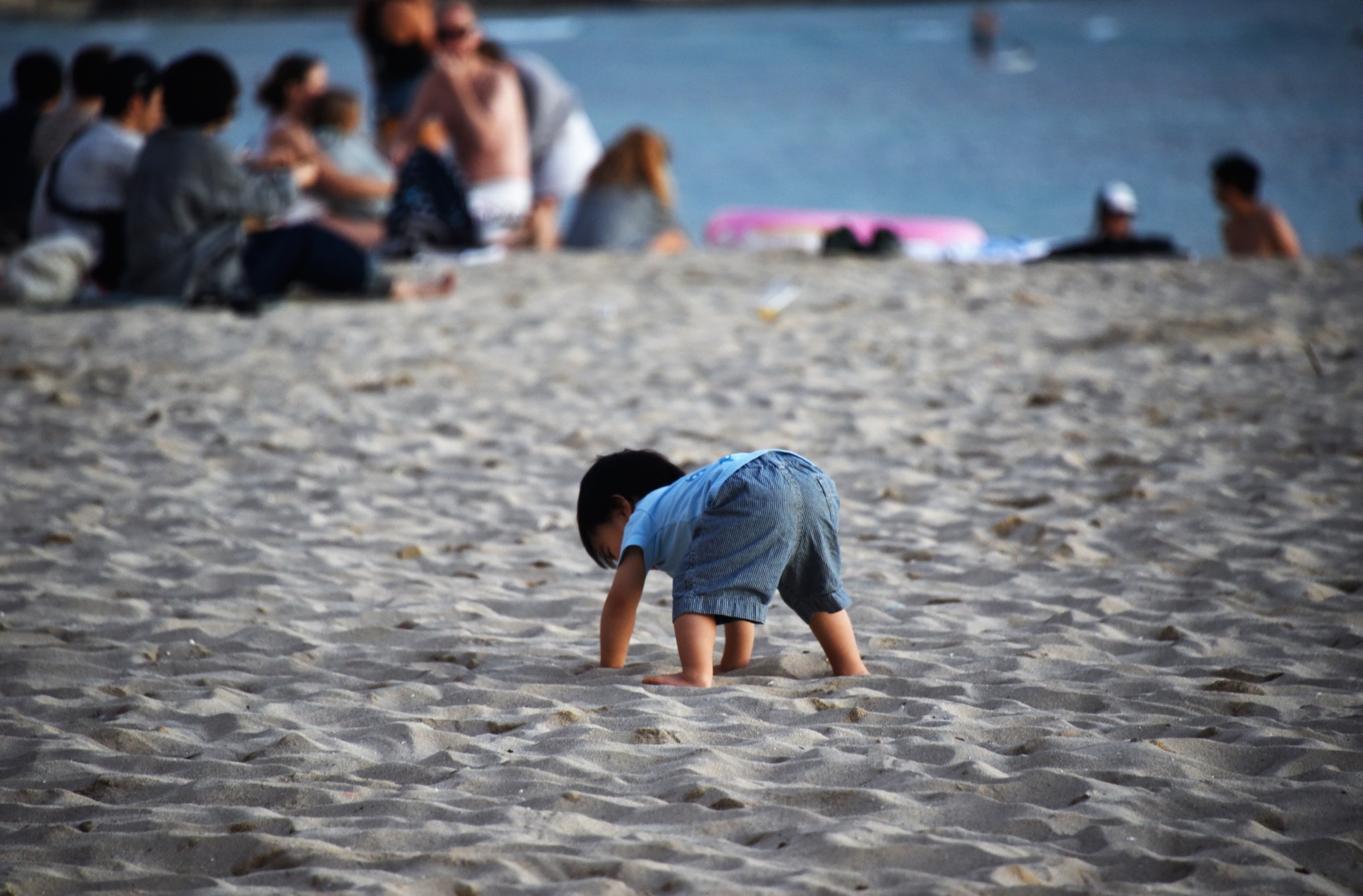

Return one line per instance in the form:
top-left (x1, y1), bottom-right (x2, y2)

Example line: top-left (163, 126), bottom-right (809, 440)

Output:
top-left (436, 26), bottom-right (470, 41)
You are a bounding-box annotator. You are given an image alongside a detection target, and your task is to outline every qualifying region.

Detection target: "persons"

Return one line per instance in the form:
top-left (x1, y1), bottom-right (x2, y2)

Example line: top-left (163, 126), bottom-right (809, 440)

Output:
top-left (1203, 146), bottom-right (1308, 260)
top-left (22, 46), bottom-right (175, 289)
top-left (29, 35), bottom-right (121, 196)
top-left (0, 47), bottom-right (69, 266)
top-left (1021, 177), bottom-right (1196, 268)
top-left (248, 0), bottom-right (932, 315)
top-left (571, 447), bottom-right (873, 689)
top-left (113, 46), bottom-right (468, 318)
top-left (964, 9), bottom-right (1029, 68)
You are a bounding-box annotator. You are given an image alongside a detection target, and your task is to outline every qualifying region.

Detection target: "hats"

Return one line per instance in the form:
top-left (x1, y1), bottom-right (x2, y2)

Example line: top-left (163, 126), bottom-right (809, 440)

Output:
top-left (1094, 179), bottom-right (1140, 220)
